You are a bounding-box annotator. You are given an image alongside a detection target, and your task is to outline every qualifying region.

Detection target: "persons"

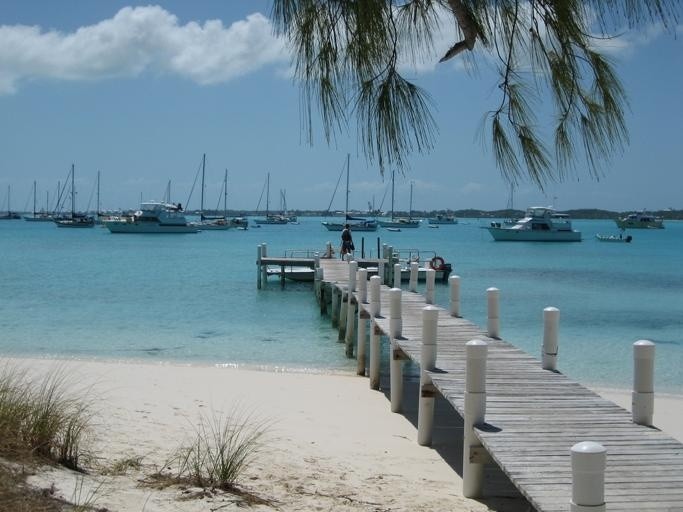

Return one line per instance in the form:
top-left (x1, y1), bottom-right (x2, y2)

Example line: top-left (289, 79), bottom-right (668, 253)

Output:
top-left (341, 223), bottom-right (352, 261)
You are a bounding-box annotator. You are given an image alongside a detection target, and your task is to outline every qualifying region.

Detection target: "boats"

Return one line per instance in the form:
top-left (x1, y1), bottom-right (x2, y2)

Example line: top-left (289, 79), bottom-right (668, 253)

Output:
top-left (479, 182), bottom-right (582, 241)
top-left (596, 208), bottom-right (665, 243)
top-left (267, 237), bottom-right (453, 282)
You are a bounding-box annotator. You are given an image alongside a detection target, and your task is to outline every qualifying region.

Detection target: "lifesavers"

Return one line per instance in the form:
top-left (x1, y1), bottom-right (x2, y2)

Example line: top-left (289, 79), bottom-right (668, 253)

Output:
top-left (430, 257), bottom-right (445, 270)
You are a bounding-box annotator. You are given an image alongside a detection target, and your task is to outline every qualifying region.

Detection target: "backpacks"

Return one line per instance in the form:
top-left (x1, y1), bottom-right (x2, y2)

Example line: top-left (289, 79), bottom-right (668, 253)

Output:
top-left (344, 230), bottom-right (349, 241)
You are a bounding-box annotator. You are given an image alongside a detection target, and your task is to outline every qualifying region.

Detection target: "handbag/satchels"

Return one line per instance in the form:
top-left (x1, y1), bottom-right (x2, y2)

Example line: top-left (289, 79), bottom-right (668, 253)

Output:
top-left (350, 242), bottom-right (355, 250)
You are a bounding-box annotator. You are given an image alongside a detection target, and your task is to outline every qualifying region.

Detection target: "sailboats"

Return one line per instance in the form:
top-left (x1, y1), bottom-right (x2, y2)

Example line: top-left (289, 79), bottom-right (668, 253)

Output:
top-left (0, 153), bottom-right (299, 234)
top-left (321, 153), bottom-right (458, 232)
